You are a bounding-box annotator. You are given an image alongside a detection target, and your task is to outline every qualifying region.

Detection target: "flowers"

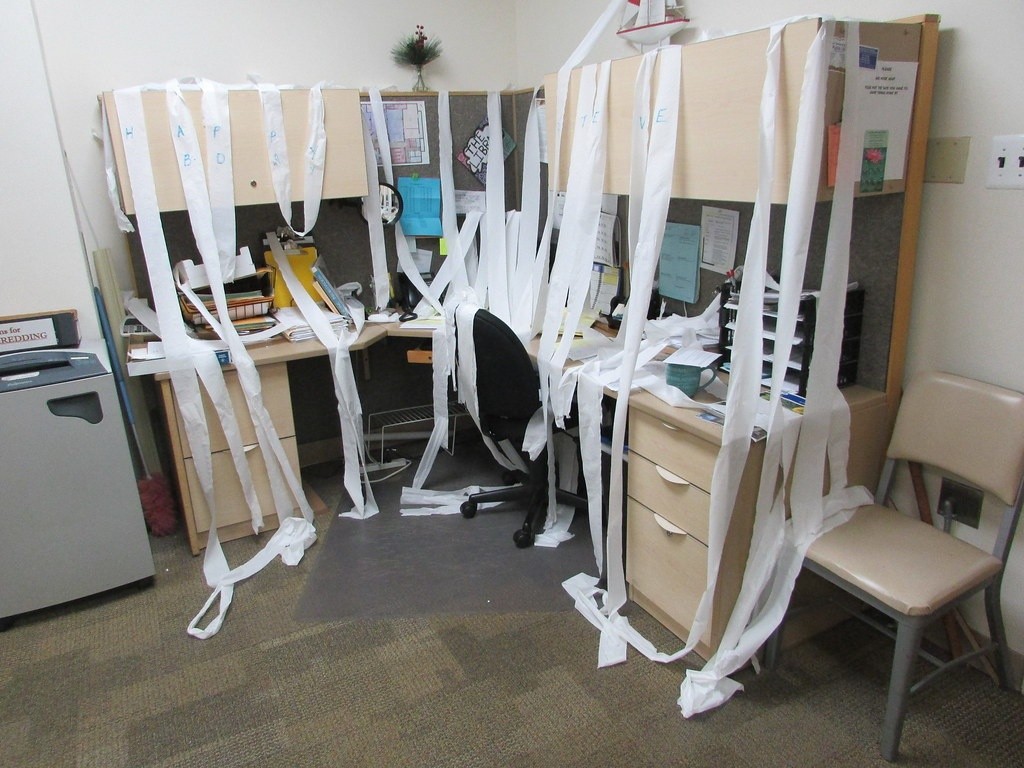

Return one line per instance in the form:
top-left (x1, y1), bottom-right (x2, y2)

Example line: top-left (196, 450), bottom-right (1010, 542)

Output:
top-left (389, 25), bottom-right (443, 69)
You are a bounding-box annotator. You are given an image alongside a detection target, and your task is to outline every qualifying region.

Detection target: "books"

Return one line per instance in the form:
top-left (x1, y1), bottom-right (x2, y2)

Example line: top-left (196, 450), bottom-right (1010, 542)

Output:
top-left (268, 301), bottom-right (349, 344)
top-left (264, 247), bottom-right (354, 326)
top-left (601, 435), bottom-right (628, 463)
top-left (539, 262), bottom-right (625, 336)
top-left (179, 289), bottom-right (277, 340)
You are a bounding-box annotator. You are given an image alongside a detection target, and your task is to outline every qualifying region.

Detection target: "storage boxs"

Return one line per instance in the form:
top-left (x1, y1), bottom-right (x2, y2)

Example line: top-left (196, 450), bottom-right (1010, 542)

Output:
top-left (126, 332), bottom-right (233, 377)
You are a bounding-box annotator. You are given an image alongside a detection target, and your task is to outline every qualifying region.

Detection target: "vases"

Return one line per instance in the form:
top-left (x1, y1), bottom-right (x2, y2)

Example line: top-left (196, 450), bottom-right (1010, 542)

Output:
top-left (412, 64), bottom-right (430, 92)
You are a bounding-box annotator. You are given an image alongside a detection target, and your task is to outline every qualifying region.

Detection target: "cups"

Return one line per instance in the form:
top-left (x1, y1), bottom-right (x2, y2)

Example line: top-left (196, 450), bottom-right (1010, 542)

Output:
top-left (665, 363), bottom-right (717, 398)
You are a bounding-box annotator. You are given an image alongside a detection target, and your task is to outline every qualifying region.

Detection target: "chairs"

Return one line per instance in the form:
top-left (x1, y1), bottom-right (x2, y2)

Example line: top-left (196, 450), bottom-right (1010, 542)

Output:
top-left (458, 308), bottom-right (589, 552)
top-left (762, 371), bottom-right (1024, 762)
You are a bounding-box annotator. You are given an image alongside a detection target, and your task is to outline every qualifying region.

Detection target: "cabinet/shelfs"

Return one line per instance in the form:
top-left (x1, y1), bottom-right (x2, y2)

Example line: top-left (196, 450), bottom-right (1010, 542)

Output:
top-left (717, 282), bottom-right (866, 397)
top-left (96, 12), bottom-right (942, 660)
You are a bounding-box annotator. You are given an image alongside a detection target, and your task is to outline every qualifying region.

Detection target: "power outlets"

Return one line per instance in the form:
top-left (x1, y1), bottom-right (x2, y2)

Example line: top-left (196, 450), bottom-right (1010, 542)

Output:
top-left (936, 478), bottom-right (984, 530)
top-left (985, 133), bottom-right (1024, 190)
